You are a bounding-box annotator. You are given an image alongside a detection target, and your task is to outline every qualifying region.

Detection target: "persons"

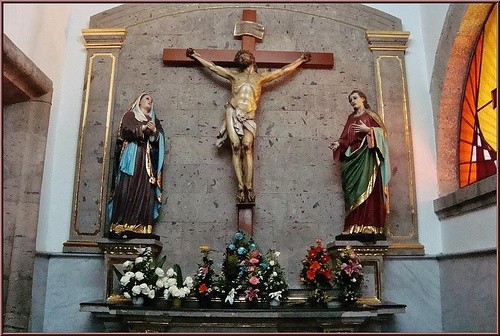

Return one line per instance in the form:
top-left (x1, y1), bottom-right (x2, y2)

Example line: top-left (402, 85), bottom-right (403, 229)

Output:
top-left (108, 92), bottom-right (165, 234)
top-left (328, 89), bottom-right (392, 238)
top-left (185, 44), bottom-right (311, 201)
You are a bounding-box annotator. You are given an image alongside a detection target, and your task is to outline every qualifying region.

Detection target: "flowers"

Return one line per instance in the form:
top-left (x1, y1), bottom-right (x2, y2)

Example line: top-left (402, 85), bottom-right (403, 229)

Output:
top-left (112, 230), bottom-right (374, 308)
